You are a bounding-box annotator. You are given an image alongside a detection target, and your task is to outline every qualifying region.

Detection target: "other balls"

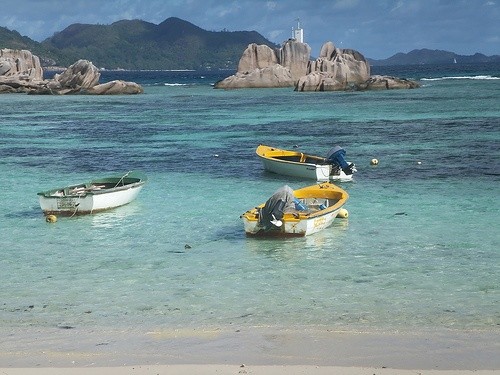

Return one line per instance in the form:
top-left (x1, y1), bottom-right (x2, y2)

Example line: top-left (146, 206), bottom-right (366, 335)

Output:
top-left (370, 158), bottom-right (379, 165)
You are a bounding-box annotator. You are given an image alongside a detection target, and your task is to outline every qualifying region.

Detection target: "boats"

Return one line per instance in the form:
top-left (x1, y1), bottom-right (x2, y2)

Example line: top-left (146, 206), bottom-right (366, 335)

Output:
top-left (35, 172), bottom-right (148, 215)
top-left (256, 144), bottom-right (355, 183)
top-left (240, 182), bottom-right (350, 237)
